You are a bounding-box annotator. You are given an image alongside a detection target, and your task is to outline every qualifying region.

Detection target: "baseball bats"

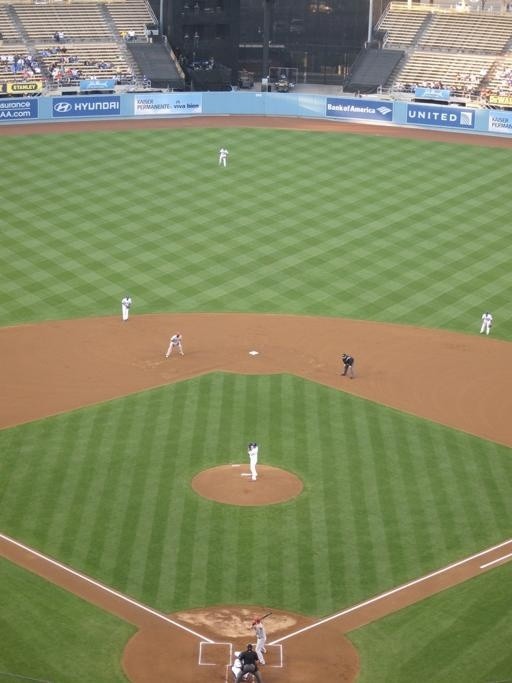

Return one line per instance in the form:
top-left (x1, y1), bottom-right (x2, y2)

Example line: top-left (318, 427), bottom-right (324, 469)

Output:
top-left (252, 613), bottom-right (272, 626)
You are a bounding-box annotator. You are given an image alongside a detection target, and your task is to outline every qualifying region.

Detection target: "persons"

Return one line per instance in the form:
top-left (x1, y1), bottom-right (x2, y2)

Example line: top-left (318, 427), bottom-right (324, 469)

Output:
top-left (120, 294), bottom-right (133, 319)
top-left (247, 440), bottom-right (260, 480)
top-left (232, 650), bottom-right (250, 682)
top-left (218, 145), bottom-right (229, 166)
top-left (341, 353), bottom-right (355, 378)
top-left (237, 642), bottom-right (262, 681)
top-left (0, 28), bottom-right (151, 90)
top-left (480, 312), bottom-right (493, 334)
top-left (165, 332), bottom-right (183, 354)
top-left (252, 617), bottom-right (269, 662)
top-left (391, 59), bottom-right (511, 110)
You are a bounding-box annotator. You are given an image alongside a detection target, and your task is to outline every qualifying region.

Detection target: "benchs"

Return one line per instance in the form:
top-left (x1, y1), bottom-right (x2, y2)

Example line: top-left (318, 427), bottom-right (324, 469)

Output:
top-left (342, 0), bottom-right (511, 106)
top-left (0, 0), bottom-right (180, 91)
